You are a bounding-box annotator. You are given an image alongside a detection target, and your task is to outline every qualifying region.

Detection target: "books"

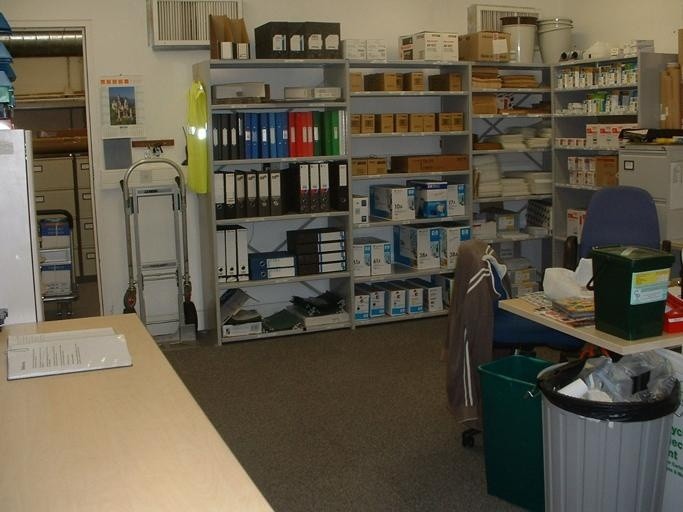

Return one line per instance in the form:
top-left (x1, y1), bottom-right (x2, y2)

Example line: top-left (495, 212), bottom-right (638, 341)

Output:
top-left (287, 305), bottom-right (350, 327)
top-left (473, 124), bottom-right (550, 239)
top-left (6, 326), bottom-right (132, 381)
top-left (517, 286), bottom-right (596, 329)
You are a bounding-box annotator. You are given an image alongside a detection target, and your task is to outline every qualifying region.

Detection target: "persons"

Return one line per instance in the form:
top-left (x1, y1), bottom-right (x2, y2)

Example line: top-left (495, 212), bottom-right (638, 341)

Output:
top-left (111, 93), bottom-right (133, 122)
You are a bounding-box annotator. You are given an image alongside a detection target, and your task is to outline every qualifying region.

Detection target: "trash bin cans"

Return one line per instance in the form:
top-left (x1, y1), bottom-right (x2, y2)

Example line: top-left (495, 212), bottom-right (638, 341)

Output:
top-left (477, 355), bottom-right (558, 512)
top-left (537, 361), bottom-right (681, 511)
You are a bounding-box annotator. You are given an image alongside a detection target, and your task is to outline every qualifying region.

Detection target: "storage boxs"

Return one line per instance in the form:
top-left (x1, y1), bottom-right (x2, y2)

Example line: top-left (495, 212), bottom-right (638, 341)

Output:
top-left (351, 112), bottom-right (462, 131)
top-left (344, 30), bottom-right (460, 92)
top-left (459, 30), bottom-right (510, 62)
top-left (356, 155), bottom-right (469, 315)
top-left (37, 217), bottom-right (71, 298)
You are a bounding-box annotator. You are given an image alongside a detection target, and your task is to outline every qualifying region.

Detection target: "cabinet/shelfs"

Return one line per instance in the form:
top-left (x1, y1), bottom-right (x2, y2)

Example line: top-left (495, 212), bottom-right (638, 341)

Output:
top-left (33, 157), bottom-right (80, 277)
top-left (10, 55), bottom-right (85, 112)
top-left (348, 60), bottom-right (471, 331)
top-left (552, 52), bottom-right (676, 272)
top-left (191, 59), bottom-right (346, 345)
top-left (472, 64), bottom-right (552, 292)
top-left (75, 155), bottom-right (98, 278)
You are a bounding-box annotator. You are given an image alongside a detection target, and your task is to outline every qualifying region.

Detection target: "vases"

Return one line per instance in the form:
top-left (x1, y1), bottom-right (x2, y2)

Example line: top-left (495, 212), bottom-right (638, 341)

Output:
top-left (499, 14), bottom-right (573, 66)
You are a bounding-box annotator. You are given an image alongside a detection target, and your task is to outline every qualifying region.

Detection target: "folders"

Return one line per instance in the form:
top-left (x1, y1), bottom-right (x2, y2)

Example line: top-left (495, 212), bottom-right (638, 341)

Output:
top-left (298, 163), bottom-right (348, 214)
top-left (285, 228), bottom-right (346, 276)
top-left (208, 110), bottom-right (343, 158)
top-left (250, 251), bottom-right (296, 280)
top-left (212, 170), bottom-right (281, 220)
top-left (216, 225), bottom-right (250, 284)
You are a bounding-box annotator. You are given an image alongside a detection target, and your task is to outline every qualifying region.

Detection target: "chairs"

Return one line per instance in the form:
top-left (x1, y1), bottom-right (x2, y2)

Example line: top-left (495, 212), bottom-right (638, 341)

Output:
top-left (561, 184), bottom-right (672, 272)
top-left (442, 239), bottom-right (580, 460)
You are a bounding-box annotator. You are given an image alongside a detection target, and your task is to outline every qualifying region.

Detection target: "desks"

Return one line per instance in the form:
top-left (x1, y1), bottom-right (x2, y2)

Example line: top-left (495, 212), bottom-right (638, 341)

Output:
top-left (496, 281), bottom-right (682, 356)
top-left (0, 314), bottom-right (275, 512)
